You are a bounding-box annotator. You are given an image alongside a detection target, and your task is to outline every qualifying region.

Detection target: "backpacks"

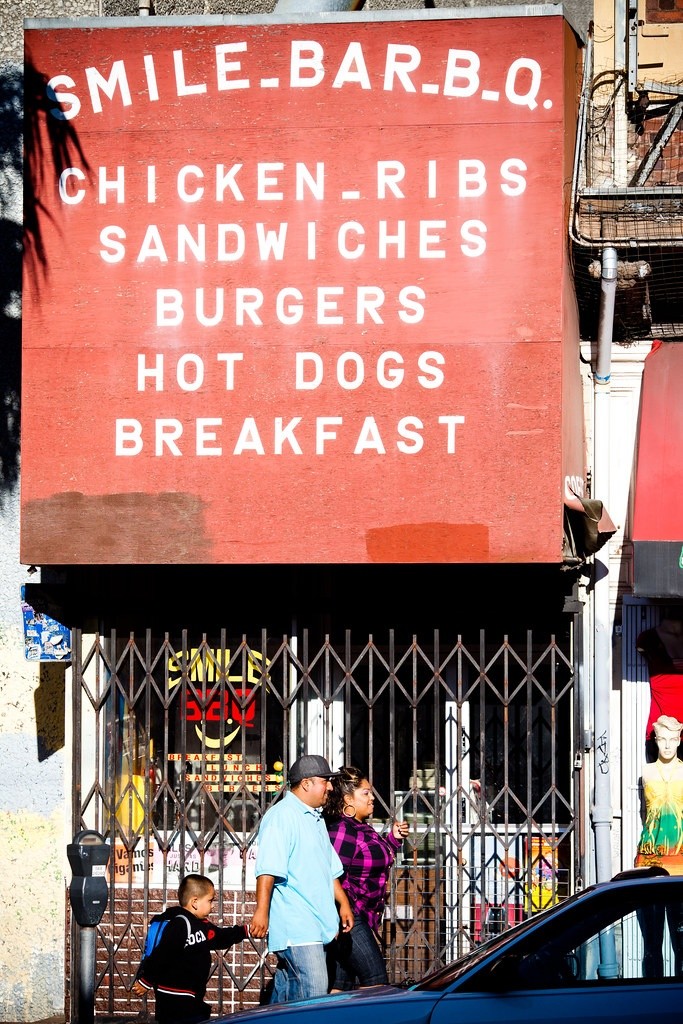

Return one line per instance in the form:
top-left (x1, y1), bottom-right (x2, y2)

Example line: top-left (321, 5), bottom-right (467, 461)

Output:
top-left (136, 914), bottom-right (191, 961)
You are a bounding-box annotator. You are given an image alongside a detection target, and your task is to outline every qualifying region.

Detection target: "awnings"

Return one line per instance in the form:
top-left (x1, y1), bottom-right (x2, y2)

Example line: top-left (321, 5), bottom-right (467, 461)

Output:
top-left (627, 343), bottom-right (683, 541)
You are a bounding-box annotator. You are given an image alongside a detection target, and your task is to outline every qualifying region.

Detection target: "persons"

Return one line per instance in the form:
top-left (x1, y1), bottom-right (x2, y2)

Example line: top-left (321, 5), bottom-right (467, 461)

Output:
top-left (635, 606), bottom-right (683, 875)
top-left (249, 755), bottom-right (355, 1004)
top-left (134, 874), bottom-right (269, 1024)
top-left (322, 769), bottom-right (409, 995)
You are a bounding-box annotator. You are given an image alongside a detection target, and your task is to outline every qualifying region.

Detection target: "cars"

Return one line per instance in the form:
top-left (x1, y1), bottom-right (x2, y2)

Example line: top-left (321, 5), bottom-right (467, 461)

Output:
top-left (203, 864), bottom-right (683, 1023)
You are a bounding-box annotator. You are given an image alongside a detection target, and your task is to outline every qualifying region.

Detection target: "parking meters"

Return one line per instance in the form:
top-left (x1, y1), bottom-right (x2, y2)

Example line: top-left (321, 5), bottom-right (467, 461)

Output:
top-left (67, 829), bottom-right (110, 1023)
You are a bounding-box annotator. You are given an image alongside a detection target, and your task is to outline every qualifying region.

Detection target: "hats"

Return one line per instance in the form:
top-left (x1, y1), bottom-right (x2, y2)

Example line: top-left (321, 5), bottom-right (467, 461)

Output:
top-left (288, 755), bottom-right (340, 784)
